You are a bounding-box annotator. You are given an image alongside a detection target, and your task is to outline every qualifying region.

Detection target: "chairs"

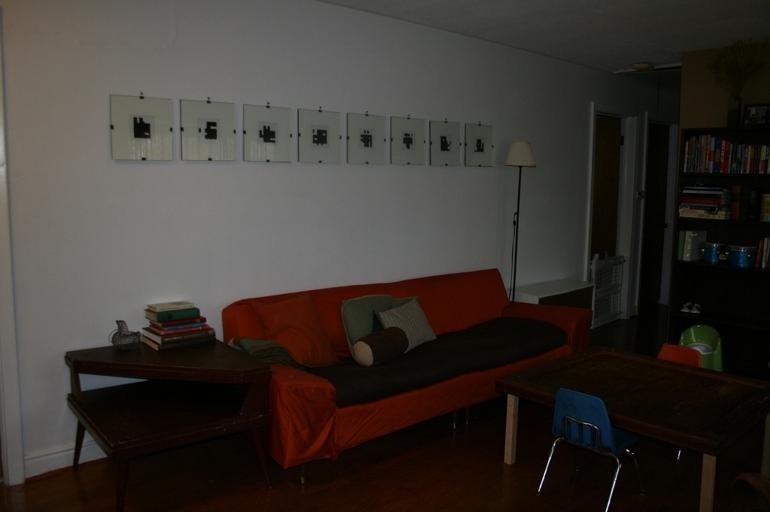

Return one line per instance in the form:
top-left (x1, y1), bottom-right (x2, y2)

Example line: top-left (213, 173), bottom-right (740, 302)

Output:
top-left (538, 388), bottom-right (648, 510)
top-left (658, 345), bottom-right (701, 461)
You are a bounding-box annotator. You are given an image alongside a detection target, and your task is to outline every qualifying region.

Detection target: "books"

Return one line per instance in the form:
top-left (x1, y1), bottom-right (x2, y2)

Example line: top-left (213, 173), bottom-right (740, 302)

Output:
top-left (677, 229), bottom-right (707, 261)
top-left (140, 301), bottom-right (216, 351)
top-left (756, 236), bottom-right (770, 269)
top-left (678, 184), bottom-right (770, 223)
top-left (682, 132), bottom-right (770, 174)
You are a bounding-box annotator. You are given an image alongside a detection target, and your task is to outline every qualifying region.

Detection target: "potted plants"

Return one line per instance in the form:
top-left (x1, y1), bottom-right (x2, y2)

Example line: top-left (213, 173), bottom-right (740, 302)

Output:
top-left (708, 34), bottom-right (755, 129)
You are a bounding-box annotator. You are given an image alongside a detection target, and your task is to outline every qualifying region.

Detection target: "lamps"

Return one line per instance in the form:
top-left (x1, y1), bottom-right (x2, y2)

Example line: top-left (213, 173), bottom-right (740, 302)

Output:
top-left (504, 139), bottom-right (539, 307)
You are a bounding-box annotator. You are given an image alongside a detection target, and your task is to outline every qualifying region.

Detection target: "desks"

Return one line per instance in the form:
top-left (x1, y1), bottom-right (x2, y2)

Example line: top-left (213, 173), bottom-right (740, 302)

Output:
top-left (66, 341), bottom-right (267, 512)
top-left (496, 347), bottom-right (770, 511)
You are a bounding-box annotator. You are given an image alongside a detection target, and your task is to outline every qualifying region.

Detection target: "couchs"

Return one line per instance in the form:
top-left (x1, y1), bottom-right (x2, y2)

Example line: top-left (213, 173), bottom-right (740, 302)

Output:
top-left (222, 269), bottom-right (592, 474)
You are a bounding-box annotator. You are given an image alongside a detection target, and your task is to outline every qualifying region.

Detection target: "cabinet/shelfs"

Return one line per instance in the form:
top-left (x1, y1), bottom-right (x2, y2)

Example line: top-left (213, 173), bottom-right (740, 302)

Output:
top-left (669, 126), bottom-right (770, 379)
top-left (514, 280), bottom-right (594, 308)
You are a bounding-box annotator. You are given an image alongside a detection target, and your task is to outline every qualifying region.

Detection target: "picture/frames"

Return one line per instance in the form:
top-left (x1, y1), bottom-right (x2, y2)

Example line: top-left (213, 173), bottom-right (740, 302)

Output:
top-left (743, 103), bottom-right (770, 127)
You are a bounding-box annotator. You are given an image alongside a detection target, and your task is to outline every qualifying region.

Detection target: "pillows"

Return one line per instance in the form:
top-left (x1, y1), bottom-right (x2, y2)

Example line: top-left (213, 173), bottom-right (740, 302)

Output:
top-left (341, 295), bottom-right (436, 365)
